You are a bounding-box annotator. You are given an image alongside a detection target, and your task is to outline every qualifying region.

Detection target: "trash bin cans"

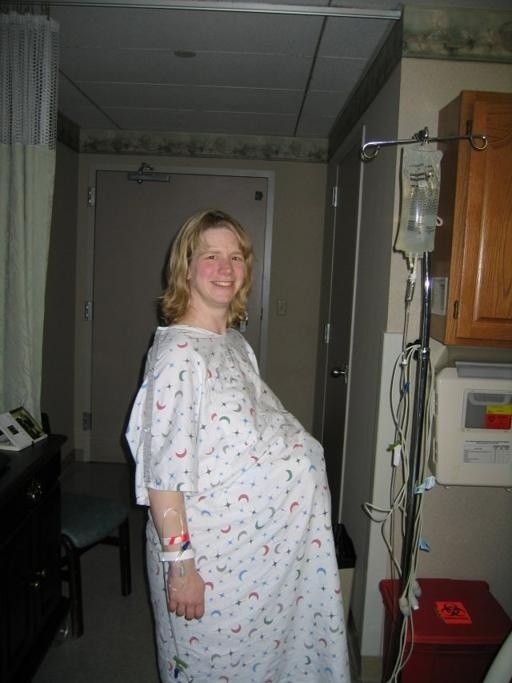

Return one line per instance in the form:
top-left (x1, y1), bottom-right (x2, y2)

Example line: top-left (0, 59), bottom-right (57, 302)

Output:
top-left (330, 523), bottom-right (357, 630)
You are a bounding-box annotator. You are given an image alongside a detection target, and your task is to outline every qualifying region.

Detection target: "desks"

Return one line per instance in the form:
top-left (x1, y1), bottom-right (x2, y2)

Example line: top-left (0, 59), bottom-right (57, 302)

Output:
top-left (0, 434), bottom-right (69, 682)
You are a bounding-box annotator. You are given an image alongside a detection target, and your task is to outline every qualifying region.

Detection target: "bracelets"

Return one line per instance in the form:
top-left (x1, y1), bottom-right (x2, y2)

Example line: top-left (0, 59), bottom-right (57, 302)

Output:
top-left (157, 545), bottom-right (197, 563)
top-left (159, 530), bottom-right (191, 547)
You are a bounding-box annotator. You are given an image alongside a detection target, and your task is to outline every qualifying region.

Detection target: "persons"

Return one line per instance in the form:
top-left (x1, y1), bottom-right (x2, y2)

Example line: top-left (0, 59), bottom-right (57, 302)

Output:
top-left (121, 205), bottom-right (356, 682)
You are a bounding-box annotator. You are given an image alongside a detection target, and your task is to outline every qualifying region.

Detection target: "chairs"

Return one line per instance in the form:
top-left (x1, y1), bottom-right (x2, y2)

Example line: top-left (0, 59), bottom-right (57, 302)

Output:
top-left (40, 412), bottom-right (134, 639)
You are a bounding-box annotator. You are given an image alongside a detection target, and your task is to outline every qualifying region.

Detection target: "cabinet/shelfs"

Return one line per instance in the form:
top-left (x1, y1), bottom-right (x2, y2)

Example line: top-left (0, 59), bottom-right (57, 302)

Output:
top-left (426, 91), bottom-right (512, 347)
